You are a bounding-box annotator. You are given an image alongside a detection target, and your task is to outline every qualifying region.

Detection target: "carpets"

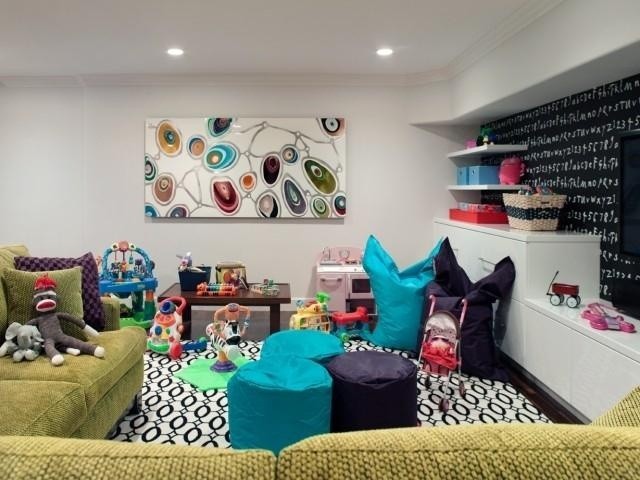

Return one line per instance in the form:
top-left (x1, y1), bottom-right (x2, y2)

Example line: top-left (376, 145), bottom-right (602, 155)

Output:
top-left (105, 337), bottom-right (554, 451)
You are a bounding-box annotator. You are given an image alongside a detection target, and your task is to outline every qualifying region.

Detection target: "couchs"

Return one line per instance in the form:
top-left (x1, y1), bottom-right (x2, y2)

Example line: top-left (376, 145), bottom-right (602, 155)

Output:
top-left (0, 244), bottom-right (640, 480)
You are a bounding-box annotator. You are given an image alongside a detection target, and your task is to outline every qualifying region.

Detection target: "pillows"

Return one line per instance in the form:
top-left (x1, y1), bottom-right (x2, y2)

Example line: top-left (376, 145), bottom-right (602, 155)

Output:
top-left (14, 251), bottom-right (107, 332)
top-left (1, 265), bottom-right (88, 343)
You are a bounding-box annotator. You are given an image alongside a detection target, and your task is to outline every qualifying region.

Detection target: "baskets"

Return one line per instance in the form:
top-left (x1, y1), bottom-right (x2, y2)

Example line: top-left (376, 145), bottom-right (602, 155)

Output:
top-left (502, 192), bottom-right (568, 230)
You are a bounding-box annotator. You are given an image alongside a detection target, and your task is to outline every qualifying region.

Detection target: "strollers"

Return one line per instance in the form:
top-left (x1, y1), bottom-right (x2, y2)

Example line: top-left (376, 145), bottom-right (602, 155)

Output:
top-left (411, 294), bottom-right (468, 411)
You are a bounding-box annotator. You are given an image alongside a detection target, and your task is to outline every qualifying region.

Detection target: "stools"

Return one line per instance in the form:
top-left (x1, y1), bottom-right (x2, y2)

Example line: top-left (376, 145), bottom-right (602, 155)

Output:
top-left (325, 350), bottom-right (417, 432)
top-left (259, 327), bottom-right (345, 361)
top-left (226, 354), bottom-right (333, 457)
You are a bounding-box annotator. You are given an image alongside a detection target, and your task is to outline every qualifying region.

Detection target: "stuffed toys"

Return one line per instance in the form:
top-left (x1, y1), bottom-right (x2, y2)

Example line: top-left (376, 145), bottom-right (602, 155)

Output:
top-left (0, 272), bottom-right (105, 366)
top-left (498, 157), bottom-right (526, 186)
top-left (466, 126), bottom-right (499, 147)
top-left (5, 320), bottom-right (44, 362)
top-left (518, 182), bottom-right (555, 198)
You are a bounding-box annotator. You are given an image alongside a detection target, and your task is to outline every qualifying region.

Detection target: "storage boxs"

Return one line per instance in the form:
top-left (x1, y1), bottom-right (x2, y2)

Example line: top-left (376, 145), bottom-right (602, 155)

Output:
top-left (468, 165), bottom-right (499, 184)
top-left (215, 266), bottom-right (246, 284)
top-left (178, 266), bottom-right (211, 291)
top-left (457, 167), bottom-right (468, 185)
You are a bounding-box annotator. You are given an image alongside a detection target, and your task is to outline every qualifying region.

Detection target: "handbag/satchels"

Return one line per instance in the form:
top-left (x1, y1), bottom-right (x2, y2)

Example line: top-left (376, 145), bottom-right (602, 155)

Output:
top-left (330, 246), bottom-right (362, 261)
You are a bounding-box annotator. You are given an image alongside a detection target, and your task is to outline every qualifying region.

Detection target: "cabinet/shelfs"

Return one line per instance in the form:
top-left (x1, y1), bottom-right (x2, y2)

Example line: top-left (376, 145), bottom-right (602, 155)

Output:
top-left (433, 143), bottom-right (640, 425)
top-left (315, 246), bottom-right (378, 318)
